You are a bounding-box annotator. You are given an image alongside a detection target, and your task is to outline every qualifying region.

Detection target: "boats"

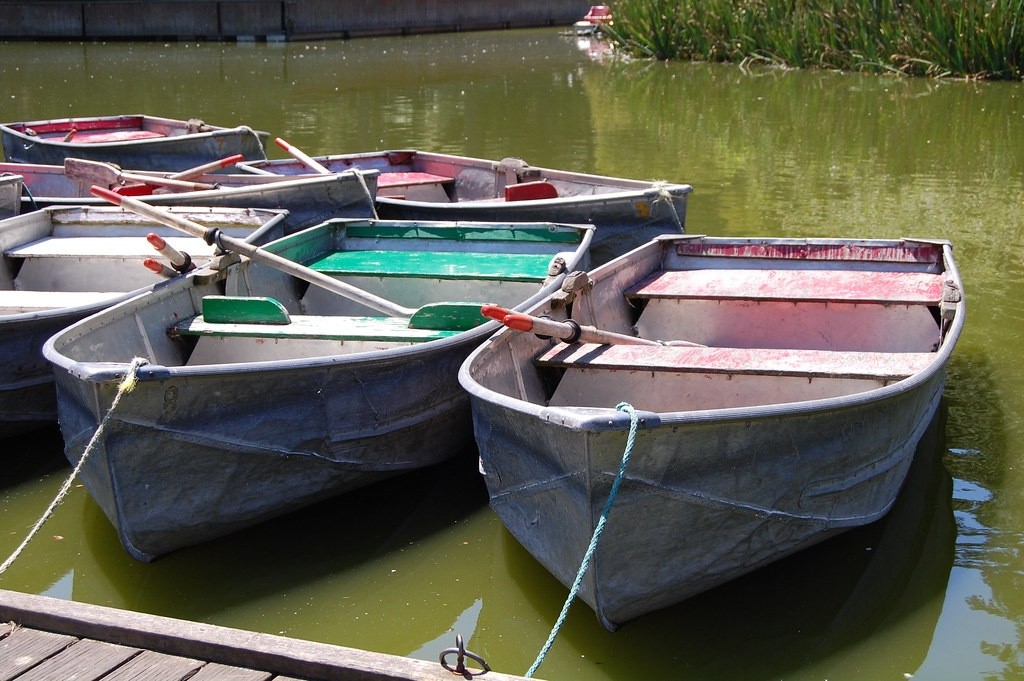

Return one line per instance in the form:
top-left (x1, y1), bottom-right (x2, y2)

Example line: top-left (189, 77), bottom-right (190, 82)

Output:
top-left (573, 5), bottom-right (611, 33)
top-left (1, 112), bottom-right (691, 562)
top-left (456, 235), bottom-right (968, 637)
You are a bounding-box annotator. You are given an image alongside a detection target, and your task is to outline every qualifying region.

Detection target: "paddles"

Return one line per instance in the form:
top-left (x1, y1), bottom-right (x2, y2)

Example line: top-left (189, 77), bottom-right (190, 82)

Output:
top-left (63, 128), bottom-right (78, 142)
top-left (151, 153), bottom-right (244, 189)
top-left (143, 233), bottom-right (198, 278)
top-left (90, 185), bottom-right (420, 317)
top-left (65, 158), bottom-right (232, 190)
top-left (479, 304), bottom-right (708, 348)
top-left (275, 137), bottom-right (332, 175)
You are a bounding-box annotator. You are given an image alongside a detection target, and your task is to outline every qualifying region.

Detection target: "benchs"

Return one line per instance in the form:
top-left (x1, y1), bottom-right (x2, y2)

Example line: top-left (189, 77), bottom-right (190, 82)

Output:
top-left (535, 341), bottom-right (938, 379)
top-left (166, 295), bottom-right (501, 343)
top-left (3, 235), bottom-right (218, 257)
top-left (377, 171), bottom-right (456, 189)
top-left (300, 250), bottom-right (556, 283)
top-left (0, 290), bottom-right (129, 310)
top-left (38, 130), bottom-right (165, 142)
top-left (625, 269), bottom-right (943, 306)
